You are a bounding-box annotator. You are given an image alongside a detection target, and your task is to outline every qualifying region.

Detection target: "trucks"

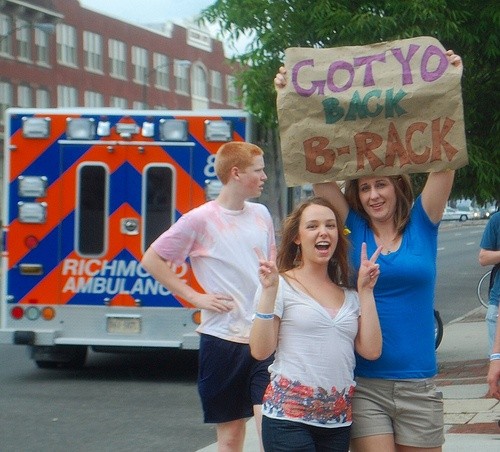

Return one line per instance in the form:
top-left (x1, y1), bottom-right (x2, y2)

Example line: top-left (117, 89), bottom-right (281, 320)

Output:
top-left (0, 106), bottom-right (314, 372)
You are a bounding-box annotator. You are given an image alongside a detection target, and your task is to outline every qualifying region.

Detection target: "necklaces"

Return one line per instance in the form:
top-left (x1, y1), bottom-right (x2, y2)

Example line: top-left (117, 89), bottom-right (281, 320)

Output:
top-left (379, 239), bottom-right (391, 254)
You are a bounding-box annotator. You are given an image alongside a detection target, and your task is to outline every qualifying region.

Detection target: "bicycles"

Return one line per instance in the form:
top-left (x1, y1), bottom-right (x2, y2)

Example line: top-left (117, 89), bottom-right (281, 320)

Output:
top-left (476, 270), bottom-right (492, 309)
top-left (434, 307), bottom-right (444, 351)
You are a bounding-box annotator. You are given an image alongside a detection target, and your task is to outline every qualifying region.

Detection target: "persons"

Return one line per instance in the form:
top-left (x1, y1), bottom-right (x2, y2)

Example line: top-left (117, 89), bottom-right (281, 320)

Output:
top-left (138, 141), bottom-right (277, 452)
top-left (249, 196), bottom-right (382, 452)
top-left (273, 48), bottom-right (465, 452)
top-left (478, 212), bottom-right (500, 401)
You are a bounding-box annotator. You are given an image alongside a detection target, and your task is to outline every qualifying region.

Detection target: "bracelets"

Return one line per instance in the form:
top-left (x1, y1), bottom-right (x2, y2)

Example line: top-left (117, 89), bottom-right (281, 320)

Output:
top-left (490, 354), bottom-right (500, 361)
top-left (254, 311), bottom-right (275, 320)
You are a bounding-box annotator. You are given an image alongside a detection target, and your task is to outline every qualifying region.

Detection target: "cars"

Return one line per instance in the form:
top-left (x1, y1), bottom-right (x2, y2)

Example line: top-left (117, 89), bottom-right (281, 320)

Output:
top-left (441, 206), bottom-right (497, 222)
